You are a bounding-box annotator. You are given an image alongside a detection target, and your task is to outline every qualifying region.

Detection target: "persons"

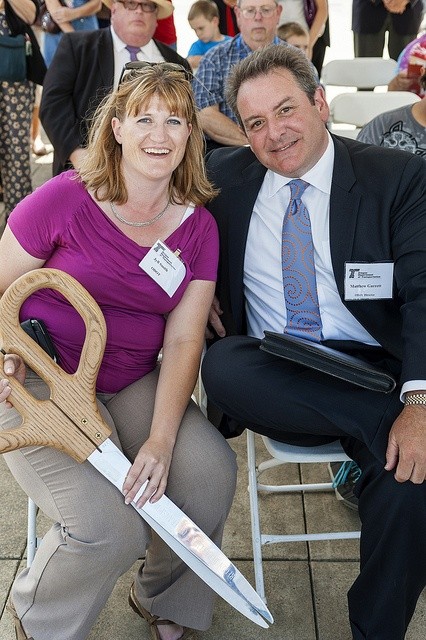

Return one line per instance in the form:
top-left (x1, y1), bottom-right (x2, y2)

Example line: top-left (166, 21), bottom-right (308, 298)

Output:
top-left (0, 1), bottom-right (48, 240)
top-left (351, 1), bottom-right (425, 129)
top-left (38, 0), bottom-right (194, 178)
top-left (277, 22), bottom-right (310, 58)
top-left (185, 0), bottom-right (234, 69)
top-left (387, 35), bottom-right (426, 99)
top-left (30, 0), bottom-right (53, 156)
top-left (325, 64), bottom-right (426, 511)
top-left (201, 43), bottom-right (426, 640)
top-left (276, 0), bottom-right (331, 81)
top-left (189, 0), bottom-right (320, 161)
top-left (154, 0), bottom-right (177, 53)
top-left (40, 0), bottom-right (102, 70)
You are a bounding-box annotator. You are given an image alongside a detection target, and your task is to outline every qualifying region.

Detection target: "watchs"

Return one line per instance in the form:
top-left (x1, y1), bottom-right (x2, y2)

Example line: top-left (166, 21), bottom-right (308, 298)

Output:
top-left (403, 393), bottom-right (426, 406)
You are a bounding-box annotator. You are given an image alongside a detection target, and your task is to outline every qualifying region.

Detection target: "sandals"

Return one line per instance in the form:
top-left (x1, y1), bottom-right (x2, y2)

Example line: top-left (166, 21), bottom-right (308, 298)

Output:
top-left (129, 582), bottom-right (200, 640)
top-left (6, 596), bottom-right (34, 640)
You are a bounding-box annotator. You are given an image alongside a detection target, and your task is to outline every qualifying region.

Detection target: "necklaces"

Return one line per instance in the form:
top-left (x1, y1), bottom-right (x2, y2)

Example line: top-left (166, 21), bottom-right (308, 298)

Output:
top-left (108, 197), bottom-right (171, 228)
top-left (0, 60), bottom-right (238, 640)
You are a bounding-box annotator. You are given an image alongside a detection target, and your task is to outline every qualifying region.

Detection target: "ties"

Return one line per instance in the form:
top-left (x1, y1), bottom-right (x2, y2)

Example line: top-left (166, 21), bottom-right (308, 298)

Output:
top-left (127, 47), bottom-right (141, 61)
top-left (281, 179), bottom-right (323, 345)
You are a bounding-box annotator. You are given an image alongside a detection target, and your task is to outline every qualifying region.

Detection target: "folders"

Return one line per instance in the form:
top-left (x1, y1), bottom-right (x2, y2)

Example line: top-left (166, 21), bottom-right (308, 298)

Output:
top-left (260, 329), bottom-right (396, 394)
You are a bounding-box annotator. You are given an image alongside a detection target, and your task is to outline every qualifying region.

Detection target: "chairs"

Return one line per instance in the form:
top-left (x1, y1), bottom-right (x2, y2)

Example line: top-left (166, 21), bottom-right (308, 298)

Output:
top-left (328, 91), bottom-right (421, 141)
top-left (245, 419), bottom-right (366, 611)
top-left (320, 58), bottom-right (400, 90)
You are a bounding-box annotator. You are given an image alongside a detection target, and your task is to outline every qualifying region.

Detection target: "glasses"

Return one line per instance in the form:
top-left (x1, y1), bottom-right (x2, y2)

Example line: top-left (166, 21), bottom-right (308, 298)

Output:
top-left (117, 61), bottom-right (188, 91)
top-left (239, 6), bottom-right (277, 17)
top-left (118, 0), bottom-right (157, 12)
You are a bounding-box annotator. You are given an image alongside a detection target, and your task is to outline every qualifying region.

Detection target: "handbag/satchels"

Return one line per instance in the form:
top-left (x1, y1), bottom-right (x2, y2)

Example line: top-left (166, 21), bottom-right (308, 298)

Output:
top-left (0, 25), bottom-right (33, 87)
top-left (41, 0), bottom-right (67, 32)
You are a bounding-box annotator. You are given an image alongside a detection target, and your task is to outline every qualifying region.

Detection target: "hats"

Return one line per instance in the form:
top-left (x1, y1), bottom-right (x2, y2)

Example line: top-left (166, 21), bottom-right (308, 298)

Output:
top-left (100, 0), bottom-right (174, 19)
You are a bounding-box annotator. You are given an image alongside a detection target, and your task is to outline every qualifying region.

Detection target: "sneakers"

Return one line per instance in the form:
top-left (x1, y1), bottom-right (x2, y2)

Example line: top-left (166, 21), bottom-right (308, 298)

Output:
top-left (328, 461), bottom-right (362, 510)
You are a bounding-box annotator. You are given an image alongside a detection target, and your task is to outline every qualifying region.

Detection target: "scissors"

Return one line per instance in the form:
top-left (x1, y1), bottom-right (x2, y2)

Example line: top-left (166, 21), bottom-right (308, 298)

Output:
top-left (0, 269), bottom-right (274, 628)
